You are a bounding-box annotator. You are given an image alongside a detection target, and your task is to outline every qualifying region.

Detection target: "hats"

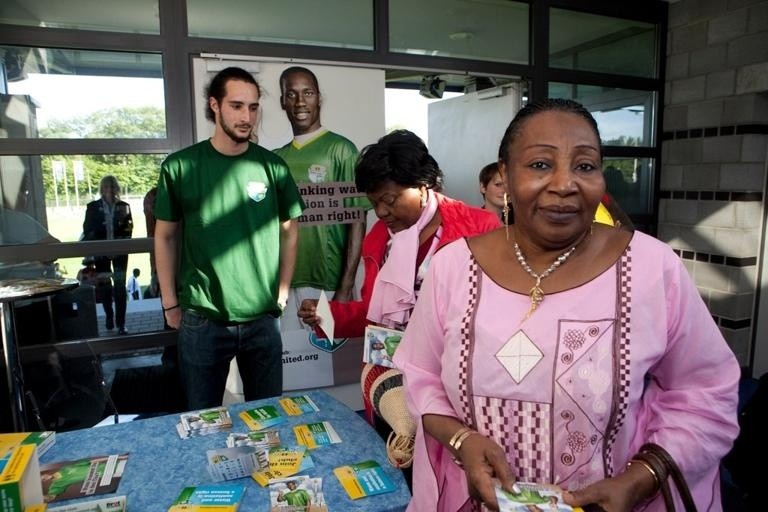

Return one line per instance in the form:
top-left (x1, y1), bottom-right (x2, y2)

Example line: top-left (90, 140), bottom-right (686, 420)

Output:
top-left (360, 363), bottom-right (416, 443)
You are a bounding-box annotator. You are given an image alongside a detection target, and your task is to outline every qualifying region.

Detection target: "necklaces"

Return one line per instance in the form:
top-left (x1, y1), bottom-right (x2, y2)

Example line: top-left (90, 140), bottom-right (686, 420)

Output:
top-left (511, 241), bottom-right (578, 323)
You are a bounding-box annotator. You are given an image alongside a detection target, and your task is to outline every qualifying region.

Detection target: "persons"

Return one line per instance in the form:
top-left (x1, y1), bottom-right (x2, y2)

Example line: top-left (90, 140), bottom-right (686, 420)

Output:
top-left (391, 96), bottom-right (744, 512)
top-left (78, 176), bottom-right (133, 335)
top-left (369, 335), bottom-right (402, 363)
top-left (291, 128), bottom-right (509, 475)
top-left (260, 66), bottom-right (381, 331)
top-left (478, 161), bottom-right (514, 226)
top-left (139, 66), bottom-right (307, 414)
top-left (500, 481), bottom-right (560, 512)
top-left (127, 267), bottom-right (143, 301)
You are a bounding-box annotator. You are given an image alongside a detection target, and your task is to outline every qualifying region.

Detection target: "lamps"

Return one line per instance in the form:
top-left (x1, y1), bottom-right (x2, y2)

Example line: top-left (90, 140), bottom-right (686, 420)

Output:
top-left (419, 72), bottom-right (496, 99)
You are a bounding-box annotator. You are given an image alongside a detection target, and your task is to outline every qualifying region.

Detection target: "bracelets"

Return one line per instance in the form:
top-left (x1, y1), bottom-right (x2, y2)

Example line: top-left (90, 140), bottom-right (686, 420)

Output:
top-left (448, 427), bottom-right (477, 469)
top-left (624, 460), bottom-right (659, 496)
top-left (161, 303), bottom-right (180, 311)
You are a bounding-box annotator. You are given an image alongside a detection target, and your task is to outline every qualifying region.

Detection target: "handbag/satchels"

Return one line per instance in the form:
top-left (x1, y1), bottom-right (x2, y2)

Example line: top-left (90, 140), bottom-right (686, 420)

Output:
top-left (76, 266), bottom-right (114, 305)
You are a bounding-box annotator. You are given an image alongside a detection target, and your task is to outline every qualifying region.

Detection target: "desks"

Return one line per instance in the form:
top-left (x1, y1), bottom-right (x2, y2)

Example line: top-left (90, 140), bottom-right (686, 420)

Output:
top-left (40, 388), bottom-right (412, 511)
top-left (0, 282), bottom-right (81, 431)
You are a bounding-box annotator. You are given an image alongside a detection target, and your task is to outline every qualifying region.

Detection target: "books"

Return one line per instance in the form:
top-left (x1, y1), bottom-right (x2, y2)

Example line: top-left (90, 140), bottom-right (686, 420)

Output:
top-left (166, 395), bottom-right (394, 512)
top-left (0, 430), bottom-right (58, 463)
top-left (492, 476), bottom-right (587, 512)
top-left (360, 325), bottom-right (404, 370)
top-left (0, 442), bottom-right (45, 512)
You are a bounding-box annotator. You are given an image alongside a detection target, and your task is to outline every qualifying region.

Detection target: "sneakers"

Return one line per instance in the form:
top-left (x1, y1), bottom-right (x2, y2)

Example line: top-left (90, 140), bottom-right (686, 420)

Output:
top-left (118, 325), bottom-right (129, 335)
top-left (106, 310), bottom-right (114, 330)
